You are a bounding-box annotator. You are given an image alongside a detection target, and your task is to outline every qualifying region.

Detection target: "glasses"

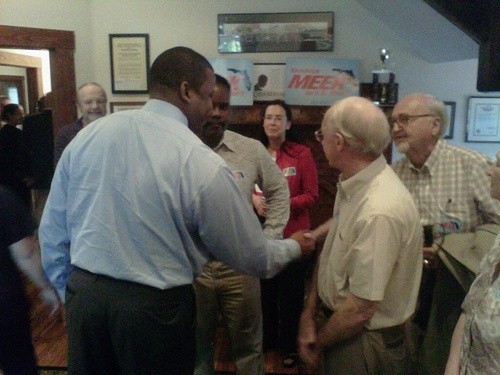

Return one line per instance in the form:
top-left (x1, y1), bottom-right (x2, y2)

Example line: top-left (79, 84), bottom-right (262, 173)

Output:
top-left (315, 128), bottom-right (338, 143)
top-left (388, 112), bottom-right (435, 127)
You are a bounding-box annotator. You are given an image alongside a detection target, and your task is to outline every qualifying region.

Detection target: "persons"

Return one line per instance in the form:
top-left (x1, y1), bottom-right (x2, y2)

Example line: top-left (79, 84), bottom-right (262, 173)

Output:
top-left (0, 75), bottom-right (500, 375)
top-left (39, 46), bottom-right (315, 375)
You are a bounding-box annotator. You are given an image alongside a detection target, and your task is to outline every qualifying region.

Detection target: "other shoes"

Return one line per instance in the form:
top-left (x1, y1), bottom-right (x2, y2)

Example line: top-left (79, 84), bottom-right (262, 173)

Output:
top-left (283, 351), bottom-right (299, 367)
